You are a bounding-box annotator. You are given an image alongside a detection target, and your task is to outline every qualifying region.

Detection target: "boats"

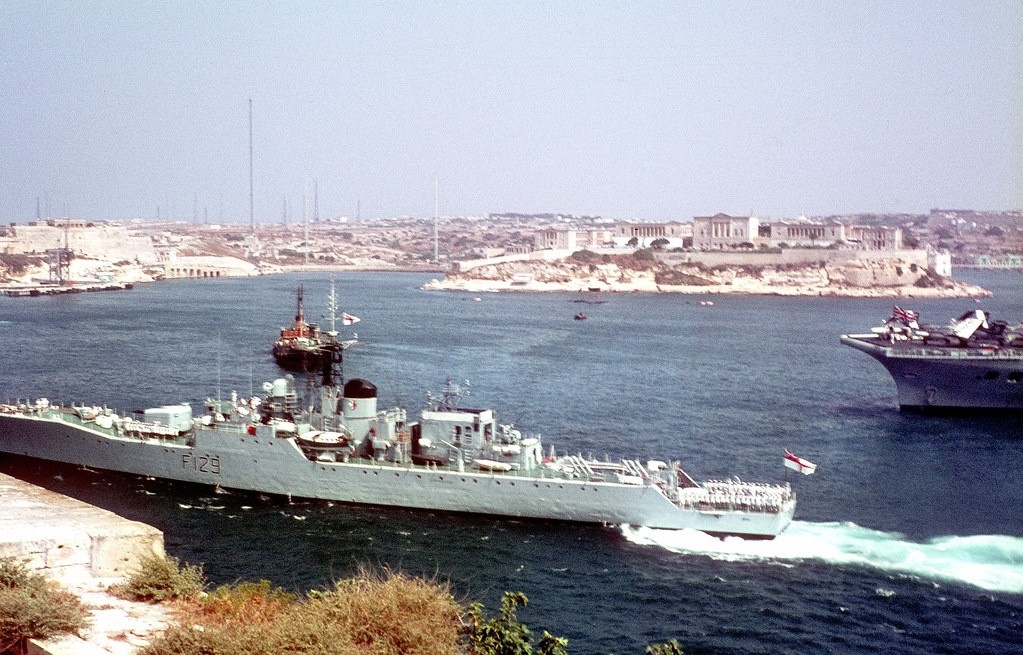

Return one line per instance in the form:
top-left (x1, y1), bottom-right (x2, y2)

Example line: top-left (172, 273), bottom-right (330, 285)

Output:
top-left (271, 282), bottom-right (328, 373)
top-left (837, 304), bottom-right (1023, 415)
top-left (2, 374), bottom-right (799, 538)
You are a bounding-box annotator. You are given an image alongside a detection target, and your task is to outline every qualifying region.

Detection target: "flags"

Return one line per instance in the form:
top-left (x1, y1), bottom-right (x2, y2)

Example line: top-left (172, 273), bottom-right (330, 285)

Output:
top-left (342, 312), bottom-right (361, 326)
top-left (782, 448), bottom-right (817, 475)
top-left (893, 304), bottom-right (914, 322)
